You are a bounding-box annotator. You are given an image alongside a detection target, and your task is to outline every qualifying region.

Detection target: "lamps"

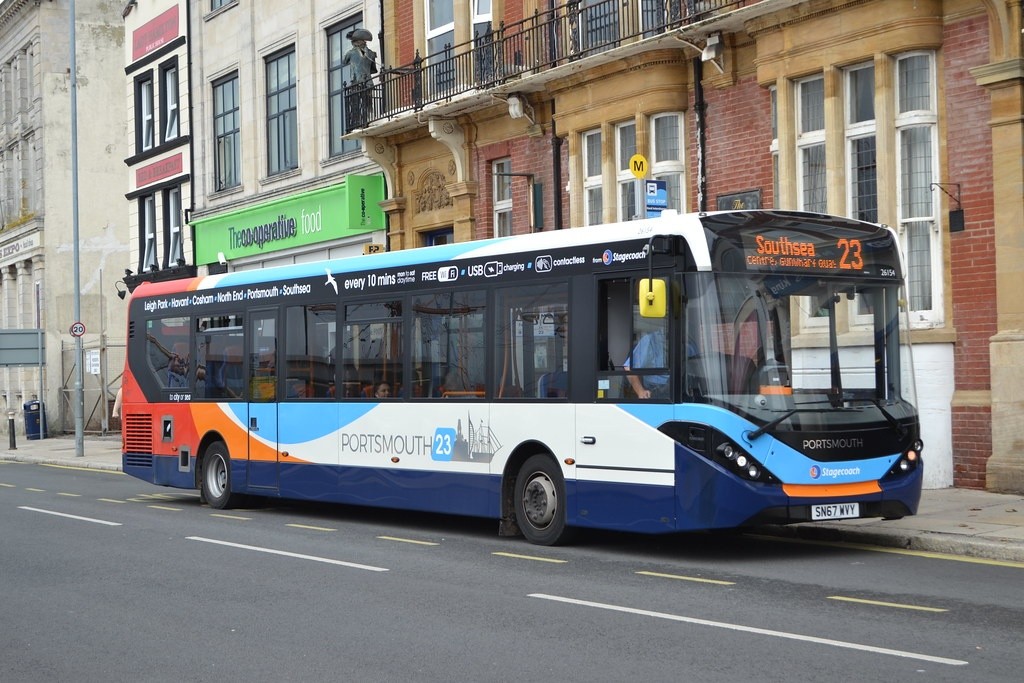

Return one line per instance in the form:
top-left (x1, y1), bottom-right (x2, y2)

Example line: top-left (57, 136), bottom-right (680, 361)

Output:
top-left (125, 268), bottom-right (134, 276)
top-left (508, 94), bottom-right (525, 120)
top-left (149, 264), bottom-right (158, 272)
top-left (115, 280), bottom-right (128, 299)
top-left (701, 30), bottom-right (726, 61)
top-left (177, 258), bottom-right (186, 268)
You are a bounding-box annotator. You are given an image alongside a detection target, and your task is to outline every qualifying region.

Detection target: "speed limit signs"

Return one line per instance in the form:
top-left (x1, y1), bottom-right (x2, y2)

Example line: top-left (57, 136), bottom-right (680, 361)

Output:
top-left (71, 322), bottom-right (85, 337)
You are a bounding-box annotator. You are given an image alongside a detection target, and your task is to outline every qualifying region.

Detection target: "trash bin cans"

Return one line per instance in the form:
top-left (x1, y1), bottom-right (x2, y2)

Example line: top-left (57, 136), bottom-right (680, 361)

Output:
top-left (24, 400), bottom-right (48, 440)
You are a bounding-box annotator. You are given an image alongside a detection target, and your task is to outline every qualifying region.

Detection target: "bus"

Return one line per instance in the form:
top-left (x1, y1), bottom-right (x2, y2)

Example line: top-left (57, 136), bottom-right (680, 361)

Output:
top-left (121, 207), bottom-right (925, 545)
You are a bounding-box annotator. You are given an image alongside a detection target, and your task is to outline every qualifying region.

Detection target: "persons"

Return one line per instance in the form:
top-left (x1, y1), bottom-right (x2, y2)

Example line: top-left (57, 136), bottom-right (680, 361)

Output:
top-left (112, 386), bottom-right (122, 421)
top-left (623, 296), bottom-right (698, 400)
top-left (342, 29), bottom-right (377, 126)
top-left (326, 338), bottom-right (391, 397)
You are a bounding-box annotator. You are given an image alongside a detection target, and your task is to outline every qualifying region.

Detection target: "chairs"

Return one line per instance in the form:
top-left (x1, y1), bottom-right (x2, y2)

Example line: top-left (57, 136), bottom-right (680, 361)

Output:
top-left (169, 344), bottom-right (422, 398)
top-left (538, 370), bottom-right (568, 398)
